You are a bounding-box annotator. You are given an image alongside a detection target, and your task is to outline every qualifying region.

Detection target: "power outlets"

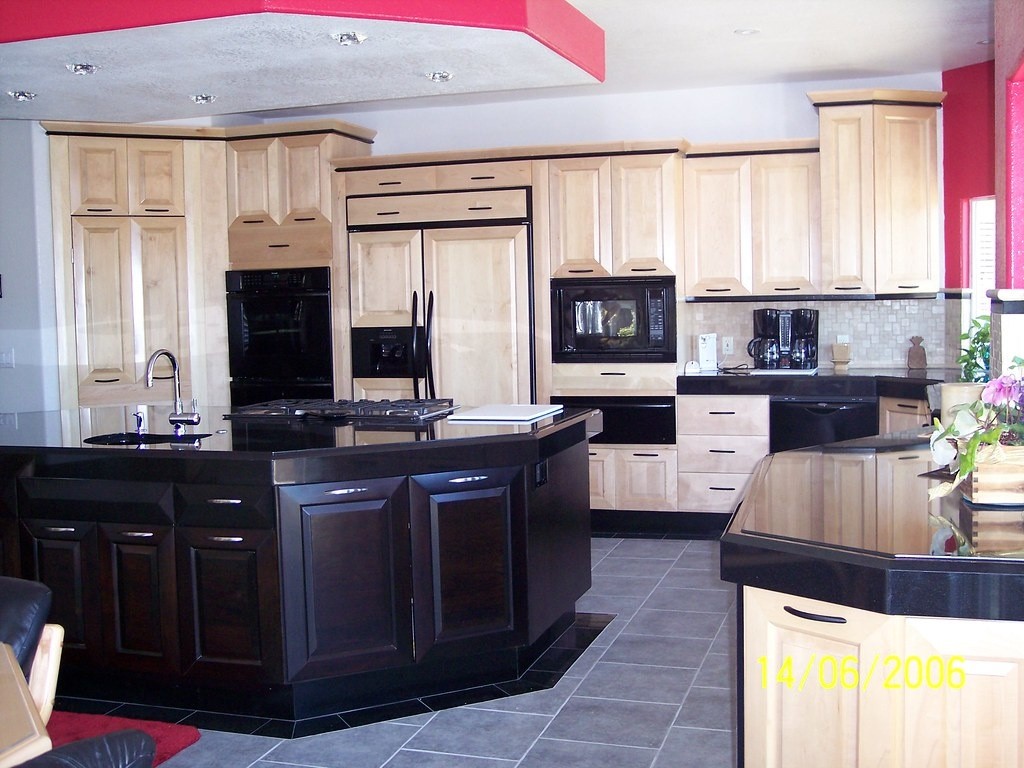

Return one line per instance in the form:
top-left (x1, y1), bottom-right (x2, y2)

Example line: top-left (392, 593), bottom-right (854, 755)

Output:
top-left (532, 459), bottom-right (551, 488)
top-left (836, 334), bottom-right (848, 343)
top-left (721, 336), bottom-right (736, 356)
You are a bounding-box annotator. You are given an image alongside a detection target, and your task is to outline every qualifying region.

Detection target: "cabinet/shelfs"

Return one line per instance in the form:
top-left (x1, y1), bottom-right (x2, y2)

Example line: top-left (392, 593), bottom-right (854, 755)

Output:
top-left (0, 477), bottom-right (173, 700)
top-left (819, 100), bottom-right (946, 294)
top-left (175, 486), bottom-right (285, 687)
top-left (684, 141), bottom-right (816, 298)
top-left (547, 148), bottom-right (677, 273)
top-left (674, 393), bottom-right (771, 515)
top-left (551, 362), bottom-right (677, 397)
top-left (580, 440), bottom-right (675, 513)
top-left (288, 469), bottom-right (519, 717)
top-left (337, 156), bottom-right (538, 443)
top-left (738, 591), bottom-right (1022, 766)
top-left (223, 123), bottom-right (342, 269)
top-left (55, 130), bottom-right (222, 405)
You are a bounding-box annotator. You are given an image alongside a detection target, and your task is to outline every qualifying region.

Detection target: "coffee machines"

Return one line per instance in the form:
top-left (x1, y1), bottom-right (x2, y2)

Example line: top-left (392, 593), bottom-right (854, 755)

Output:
top-left (748, 308), bottom-right (819, 370)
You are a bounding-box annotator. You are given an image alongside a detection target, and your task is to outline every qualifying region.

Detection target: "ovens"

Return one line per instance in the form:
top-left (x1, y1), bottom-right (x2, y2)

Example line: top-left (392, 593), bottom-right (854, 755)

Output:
top-left (225, 266), bottom-right (334, 406)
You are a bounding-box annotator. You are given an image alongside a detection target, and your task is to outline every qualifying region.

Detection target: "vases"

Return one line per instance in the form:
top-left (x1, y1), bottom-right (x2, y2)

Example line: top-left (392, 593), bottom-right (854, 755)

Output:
top-left (958, 433), bottom-right (1022, 508)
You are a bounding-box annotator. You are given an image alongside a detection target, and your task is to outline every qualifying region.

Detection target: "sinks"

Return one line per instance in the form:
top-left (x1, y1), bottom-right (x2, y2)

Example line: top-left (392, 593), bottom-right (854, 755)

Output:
top-left (83, 432), bottom-right (212, 445)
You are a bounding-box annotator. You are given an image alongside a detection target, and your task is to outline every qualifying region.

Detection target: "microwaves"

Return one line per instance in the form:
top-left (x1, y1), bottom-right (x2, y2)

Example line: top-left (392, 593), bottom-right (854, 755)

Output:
top-left (550, 275), bottom-right (678, 364)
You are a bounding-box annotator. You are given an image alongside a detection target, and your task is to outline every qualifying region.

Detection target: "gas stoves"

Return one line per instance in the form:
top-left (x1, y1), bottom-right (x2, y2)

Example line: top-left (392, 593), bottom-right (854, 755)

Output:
top-left (222, 398), bottom-right (462, 426)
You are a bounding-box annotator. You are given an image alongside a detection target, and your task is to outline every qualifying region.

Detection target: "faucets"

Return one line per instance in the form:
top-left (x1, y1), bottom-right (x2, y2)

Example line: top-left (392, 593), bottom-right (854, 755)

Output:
top-left (146, 349), bottom-right (201, 436)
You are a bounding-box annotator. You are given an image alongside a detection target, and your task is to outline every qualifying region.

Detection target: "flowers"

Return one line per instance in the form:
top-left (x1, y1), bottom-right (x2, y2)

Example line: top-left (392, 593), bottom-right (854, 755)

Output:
top-left (928, 318), bottom-right (1022, 467)
